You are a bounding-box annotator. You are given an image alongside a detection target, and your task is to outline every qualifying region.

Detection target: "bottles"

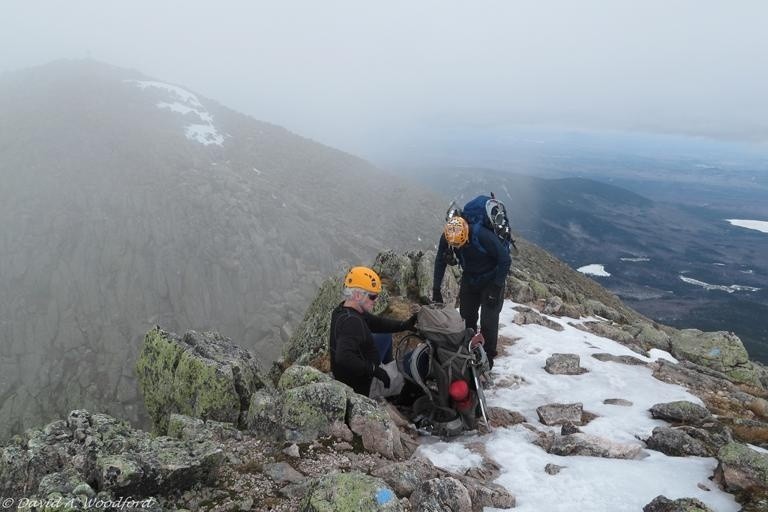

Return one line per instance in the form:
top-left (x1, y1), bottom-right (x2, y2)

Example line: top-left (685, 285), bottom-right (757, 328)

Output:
top-left (448, 380), bottom-right (475, 412)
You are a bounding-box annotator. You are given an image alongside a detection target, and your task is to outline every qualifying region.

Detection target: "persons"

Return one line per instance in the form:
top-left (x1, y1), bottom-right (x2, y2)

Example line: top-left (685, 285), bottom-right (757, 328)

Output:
top-left (433, 217), bottom-right (512, 371)
top-left (330, 266), bottom-right (432, 405)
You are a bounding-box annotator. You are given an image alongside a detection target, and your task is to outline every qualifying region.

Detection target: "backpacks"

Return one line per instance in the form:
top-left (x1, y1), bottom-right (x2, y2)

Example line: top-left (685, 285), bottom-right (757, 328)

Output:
top-left (395, 303), bottom-right (496, 442)
top-left (461, 195), bottom-right (511, 255)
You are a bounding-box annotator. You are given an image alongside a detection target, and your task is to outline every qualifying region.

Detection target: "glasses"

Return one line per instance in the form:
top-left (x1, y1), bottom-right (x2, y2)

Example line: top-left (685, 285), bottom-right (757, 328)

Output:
top-left (366, 293), bottom-right (377, 301)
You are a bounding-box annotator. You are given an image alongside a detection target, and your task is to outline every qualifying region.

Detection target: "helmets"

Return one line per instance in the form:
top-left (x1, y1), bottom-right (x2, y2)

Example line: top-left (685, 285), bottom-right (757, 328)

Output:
top-left (344, 266), bottom-right (382, 293)
top-left (443, 216), bottom-right (469, 248)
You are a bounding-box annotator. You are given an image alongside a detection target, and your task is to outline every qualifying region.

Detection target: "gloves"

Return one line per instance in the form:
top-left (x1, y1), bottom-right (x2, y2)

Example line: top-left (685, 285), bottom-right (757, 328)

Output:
top-left (432, 289), bottom-right (443, 303)
top-left (373, 367), bottom-right (390, 388)
top-left (487, 285), bottom-right (499, 305)
top-left (402, 315), bottom-right (418, 332)
